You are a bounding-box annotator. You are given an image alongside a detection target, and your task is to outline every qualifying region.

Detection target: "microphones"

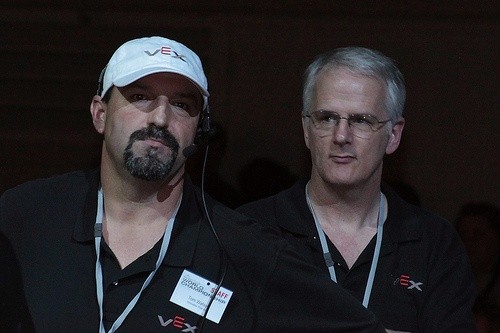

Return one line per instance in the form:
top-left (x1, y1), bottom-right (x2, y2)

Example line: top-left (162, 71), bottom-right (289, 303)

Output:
top-left (182, 143), bottom-right (197, 157)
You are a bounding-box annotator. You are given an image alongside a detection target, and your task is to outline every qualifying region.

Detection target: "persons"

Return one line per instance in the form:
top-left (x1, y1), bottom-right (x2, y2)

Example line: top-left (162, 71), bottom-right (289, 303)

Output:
top-left (1, 35), bottom-right (389, 333)
top-left (233, 44), bottom-right (480, 333)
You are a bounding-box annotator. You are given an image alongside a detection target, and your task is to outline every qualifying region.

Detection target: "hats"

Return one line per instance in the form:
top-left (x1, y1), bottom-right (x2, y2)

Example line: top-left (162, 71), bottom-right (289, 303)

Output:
top-left (100, 35), bottom-right (210, 110)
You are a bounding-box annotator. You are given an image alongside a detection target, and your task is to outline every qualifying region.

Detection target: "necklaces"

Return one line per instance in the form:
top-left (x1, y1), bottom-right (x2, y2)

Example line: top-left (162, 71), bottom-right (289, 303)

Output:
top-left (304, 178), bottom-right (384, 309)
top-left (93, 183), bottom-right (183, 333)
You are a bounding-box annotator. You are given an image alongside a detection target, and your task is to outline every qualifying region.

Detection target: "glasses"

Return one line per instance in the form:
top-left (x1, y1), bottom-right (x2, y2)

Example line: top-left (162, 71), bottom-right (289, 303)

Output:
top-left (305, 110), bottom-right (394, 139)
